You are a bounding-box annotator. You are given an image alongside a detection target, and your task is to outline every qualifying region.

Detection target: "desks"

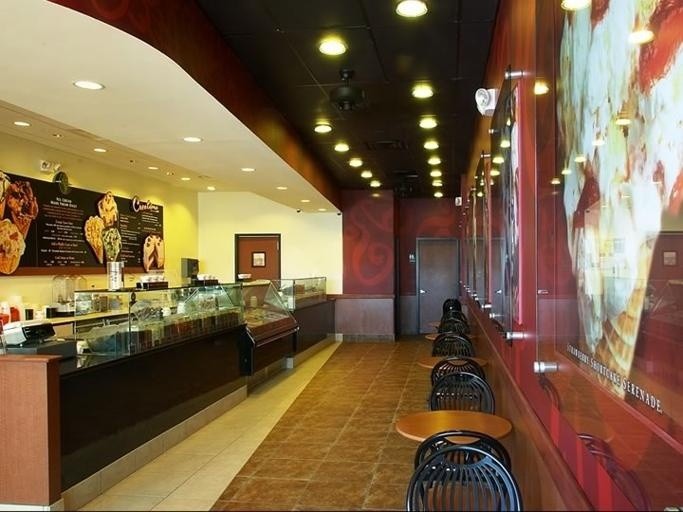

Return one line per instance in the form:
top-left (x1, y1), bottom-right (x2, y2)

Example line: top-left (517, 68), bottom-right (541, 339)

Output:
top-left (394, 409), bottom-right (513, 465)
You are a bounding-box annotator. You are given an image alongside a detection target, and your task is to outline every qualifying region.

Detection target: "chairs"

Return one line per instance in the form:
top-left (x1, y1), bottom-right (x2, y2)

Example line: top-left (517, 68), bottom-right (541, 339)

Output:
top-left (406, 298), bottom-right (524, 512)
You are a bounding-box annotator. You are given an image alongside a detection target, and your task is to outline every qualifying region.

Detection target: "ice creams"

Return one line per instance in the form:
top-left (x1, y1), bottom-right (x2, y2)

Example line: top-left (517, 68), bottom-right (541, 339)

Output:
top-left (0, 171), bottom-right (39, 275)
top-left (84, 191), bottom-right (123, 264)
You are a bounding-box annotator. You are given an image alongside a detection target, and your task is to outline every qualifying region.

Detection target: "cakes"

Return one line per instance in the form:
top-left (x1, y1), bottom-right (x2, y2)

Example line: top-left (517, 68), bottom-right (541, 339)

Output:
top-left (141, 235), bottom-right (164, 272)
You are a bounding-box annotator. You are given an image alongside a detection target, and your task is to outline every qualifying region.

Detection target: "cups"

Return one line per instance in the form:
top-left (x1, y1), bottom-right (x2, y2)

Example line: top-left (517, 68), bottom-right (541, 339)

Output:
top-left (24, 309), bottom-right (33, 320)
top-left (46, 307), bottom-right (57, 318)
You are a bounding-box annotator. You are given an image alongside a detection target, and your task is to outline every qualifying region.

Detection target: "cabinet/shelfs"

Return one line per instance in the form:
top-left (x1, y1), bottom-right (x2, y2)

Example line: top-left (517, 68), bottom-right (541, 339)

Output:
top-left (220, 282), bottom-right (300, 395)
